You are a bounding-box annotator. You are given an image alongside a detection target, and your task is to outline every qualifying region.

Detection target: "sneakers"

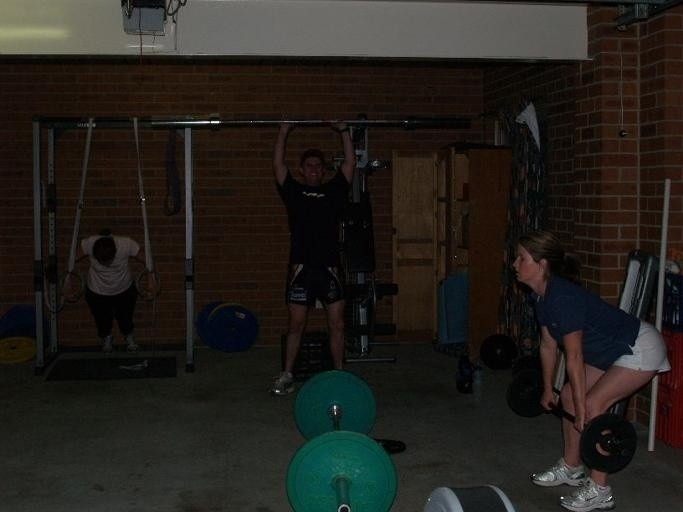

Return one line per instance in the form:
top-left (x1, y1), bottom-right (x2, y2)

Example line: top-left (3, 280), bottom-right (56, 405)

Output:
top-left (125, 334), bottom-right (139, 354)
top-left (559, 477), bottom-right (617, 512)
top-left (271, 372), bottom-right (297, 395)
top-left (529, 457), bottom-right (587, 488)
top-left (102, 334), bottom-right (114, 352)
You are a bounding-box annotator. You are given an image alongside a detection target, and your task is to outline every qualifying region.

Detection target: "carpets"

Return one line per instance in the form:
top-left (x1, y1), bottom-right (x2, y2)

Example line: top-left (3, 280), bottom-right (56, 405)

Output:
top-left (46, 355), bottom-right (178, 380)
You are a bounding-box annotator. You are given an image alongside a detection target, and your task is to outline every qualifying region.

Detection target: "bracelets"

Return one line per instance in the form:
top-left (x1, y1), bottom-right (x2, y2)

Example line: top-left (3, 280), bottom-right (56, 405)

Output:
top-left (337, 128), bottom-right (351, 133)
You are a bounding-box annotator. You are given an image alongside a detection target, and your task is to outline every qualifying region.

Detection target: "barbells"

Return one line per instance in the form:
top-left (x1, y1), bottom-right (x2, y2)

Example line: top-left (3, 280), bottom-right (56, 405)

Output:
top-left (507, 368), bottom-right (638, 472)
top-left (287, 369), bottom-right (398, 511)
top-left (480, 333), bottom-right (542, 379)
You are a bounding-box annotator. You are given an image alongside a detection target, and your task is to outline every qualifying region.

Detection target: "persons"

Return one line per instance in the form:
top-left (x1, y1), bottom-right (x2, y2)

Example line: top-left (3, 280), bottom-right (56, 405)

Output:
top-left (60, 235), bottom-right (159, 354)
top-left (269, 119), bottom-right (356, 396)
top-left (511, 230), bottom-right (669, 512)
